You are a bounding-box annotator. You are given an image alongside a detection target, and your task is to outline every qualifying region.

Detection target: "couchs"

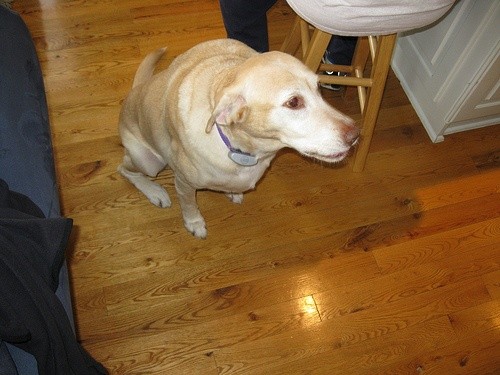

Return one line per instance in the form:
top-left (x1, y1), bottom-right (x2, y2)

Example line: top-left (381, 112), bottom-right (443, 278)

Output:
top-left (0, 6), bottom-right (108, 375)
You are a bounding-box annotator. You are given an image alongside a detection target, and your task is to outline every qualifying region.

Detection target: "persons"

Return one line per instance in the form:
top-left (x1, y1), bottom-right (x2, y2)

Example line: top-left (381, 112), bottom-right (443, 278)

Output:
top-left (219, 0), bottom-right (454, 91)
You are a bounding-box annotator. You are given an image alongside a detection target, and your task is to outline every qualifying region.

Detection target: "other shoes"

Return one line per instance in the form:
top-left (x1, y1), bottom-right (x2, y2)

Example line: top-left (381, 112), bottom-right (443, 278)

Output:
top-left (319, 50), bottom-right (347, 91)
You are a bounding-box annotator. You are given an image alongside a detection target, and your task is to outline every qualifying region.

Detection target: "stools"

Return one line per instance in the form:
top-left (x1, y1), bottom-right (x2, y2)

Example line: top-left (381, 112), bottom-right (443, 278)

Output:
top-left (279, 16), bottom-right (397, 173)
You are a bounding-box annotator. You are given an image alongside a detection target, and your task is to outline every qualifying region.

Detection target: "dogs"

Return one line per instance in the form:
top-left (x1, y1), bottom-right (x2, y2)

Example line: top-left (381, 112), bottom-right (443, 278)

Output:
top-left (117, 38), bottom-right (367, 240)
